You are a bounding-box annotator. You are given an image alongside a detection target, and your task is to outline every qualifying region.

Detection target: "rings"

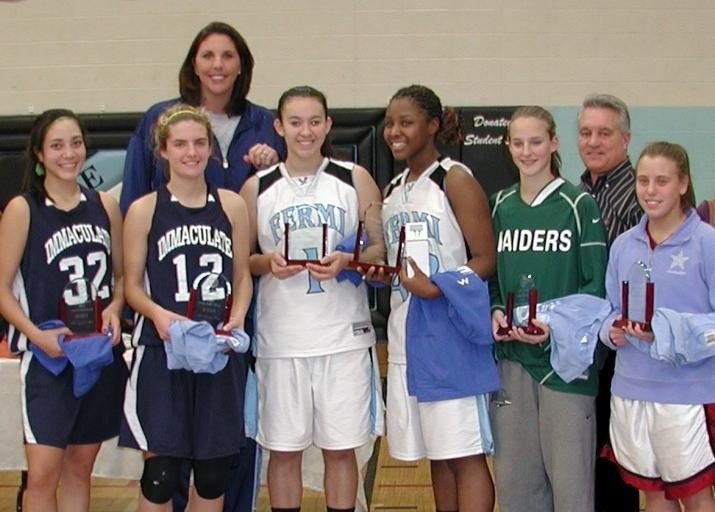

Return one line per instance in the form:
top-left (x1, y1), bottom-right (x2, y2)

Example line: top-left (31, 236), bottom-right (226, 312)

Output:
top-left (262, 150), bottom-right (269, 156)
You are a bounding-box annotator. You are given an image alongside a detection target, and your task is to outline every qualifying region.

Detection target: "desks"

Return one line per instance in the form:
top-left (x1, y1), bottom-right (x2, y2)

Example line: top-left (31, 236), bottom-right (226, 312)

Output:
top-left (0, 331), bottom-right (385, 511)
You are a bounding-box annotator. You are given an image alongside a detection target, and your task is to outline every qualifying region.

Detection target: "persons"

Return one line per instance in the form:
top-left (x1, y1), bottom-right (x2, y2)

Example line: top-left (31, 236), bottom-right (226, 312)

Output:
top-left (572, 93), bottom-right (643, 512)
top-left (597, 141), bottom-right (714, 511)
top-left (122, 20), bottom-right (286, 512)
top-left (486, 105), bottom-right (608, 510)
top-left (375, 85), bottom-right (498, 512)
top-left (0, 107), bottom-right (123, 512)
top-left (237, 85), bottom-right (385, 512)
top-left (124, 103), bottom-right (256, 512)
